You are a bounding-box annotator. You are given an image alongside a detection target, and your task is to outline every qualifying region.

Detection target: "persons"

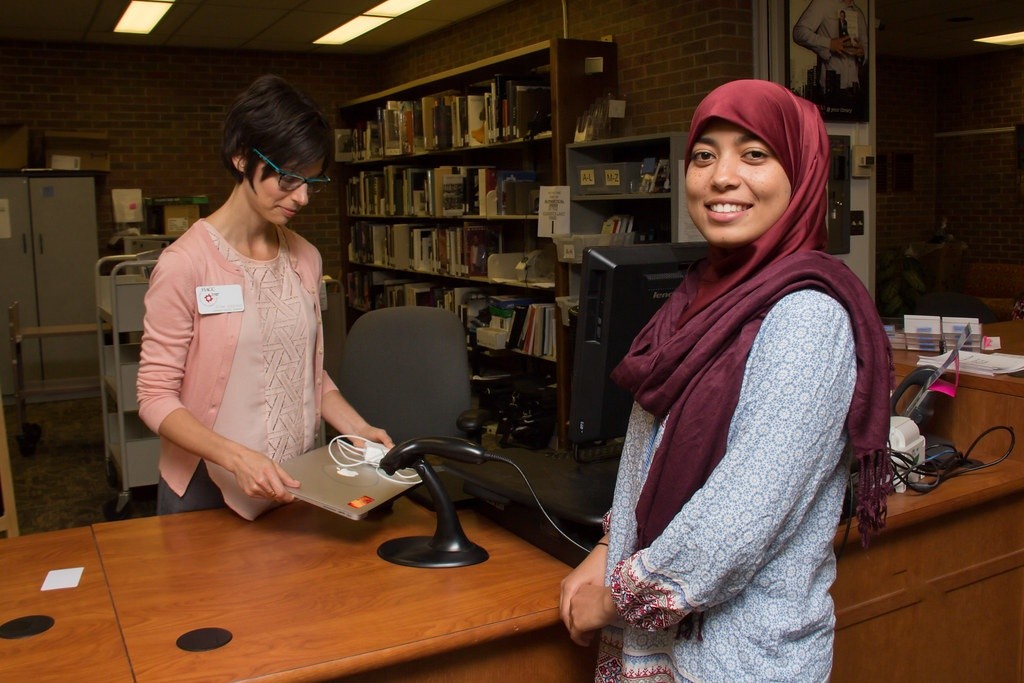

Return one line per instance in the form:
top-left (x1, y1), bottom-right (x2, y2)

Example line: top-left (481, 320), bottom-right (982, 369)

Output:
top-left (559, 79), bottom-right (893, 683)
top-left (838, 10), bottom-right (849, 37)
top-left (792, 0), bottom-right (870, 89)
top-left (137, 75), bottom-right (397, 516)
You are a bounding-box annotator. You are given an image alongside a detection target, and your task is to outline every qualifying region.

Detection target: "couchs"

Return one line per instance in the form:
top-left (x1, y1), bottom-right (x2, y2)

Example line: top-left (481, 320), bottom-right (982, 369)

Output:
top-left (964, 262), bottom-right (1024, 321)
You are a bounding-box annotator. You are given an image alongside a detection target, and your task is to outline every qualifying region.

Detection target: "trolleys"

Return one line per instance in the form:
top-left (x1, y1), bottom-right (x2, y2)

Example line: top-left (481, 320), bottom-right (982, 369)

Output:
top-left (96, 253), bottom-right (165, 520)
top-left (10, 300), bottom-right (113, 457)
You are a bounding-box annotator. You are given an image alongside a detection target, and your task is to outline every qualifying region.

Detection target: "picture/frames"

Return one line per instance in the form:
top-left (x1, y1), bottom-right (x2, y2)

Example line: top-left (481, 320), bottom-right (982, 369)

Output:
top-left (785, 0), bottom-right (870, 123)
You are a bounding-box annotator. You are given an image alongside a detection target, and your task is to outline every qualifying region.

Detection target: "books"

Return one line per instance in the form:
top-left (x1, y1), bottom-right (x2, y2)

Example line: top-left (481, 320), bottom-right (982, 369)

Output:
top-left (333, 72), bottom-right (555, 358)
top-left (597, 156), bottom-right (670, 249)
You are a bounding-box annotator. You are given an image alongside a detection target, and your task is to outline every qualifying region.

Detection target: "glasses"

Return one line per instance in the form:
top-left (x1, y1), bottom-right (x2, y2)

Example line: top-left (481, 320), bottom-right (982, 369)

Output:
top-left (253, 150), bottom-right (331, 193)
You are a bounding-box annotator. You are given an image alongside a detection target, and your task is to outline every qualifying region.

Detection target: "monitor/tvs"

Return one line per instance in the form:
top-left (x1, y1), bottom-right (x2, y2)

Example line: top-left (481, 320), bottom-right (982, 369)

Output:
top-left (568, 238), bottom-right (710, 445)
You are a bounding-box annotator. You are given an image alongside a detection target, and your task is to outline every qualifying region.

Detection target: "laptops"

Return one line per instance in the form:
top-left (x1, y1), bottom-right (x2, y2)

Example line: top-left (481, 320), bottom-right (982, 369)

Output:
top-left (275, 441), bottom-right (425, 521)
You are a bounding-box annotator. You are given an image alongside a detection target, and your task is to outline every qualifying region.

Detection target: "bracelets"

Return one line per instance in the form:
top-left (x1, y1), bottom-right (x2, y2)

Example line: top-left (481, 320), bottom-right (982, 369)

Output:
top-left (596, 542), bottom-right (608, 546)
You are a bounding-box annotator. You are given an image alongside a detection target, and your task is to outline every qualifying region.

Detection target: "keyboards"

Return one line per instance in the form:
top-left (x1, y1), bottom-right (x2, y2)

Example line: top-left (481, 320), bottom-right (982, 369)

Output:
top-left (544, 442), bottom-right (624, 463)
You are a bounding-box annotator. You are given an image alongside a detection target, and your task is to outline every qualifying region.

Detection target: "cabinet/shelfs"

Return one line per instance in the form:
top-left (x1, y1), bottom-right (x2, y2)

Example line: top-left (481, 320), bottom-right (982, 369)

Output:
top-left (566, 131), bottom-right (690, 302)
top-left (95, 254), bottom-right (161, 521)
top-left (115, 238), bottom-right (169, 343)
top-left (337, 37), bottom-right (621, 455)
top-left (905, 241), bottom-right (968, 291)
top-left (0, 167), bottom-right (109, 406)
top-left (9, 300), bottom-right (113, 457)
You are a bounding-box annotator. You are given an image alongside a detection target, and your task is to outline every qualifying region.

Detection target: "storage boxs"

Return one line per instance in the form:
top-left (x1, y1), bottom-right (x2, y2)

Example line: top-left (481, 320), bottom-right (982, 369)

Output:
top-left (556, 296), bottom-right (580, 326)
top-left (549, 231), bottom-right (635, 264)
top-left (0, 119), bottom-right (29, 168)
top-left (576, 162), bottom-right (644, 195)
top-left (162, 204), bottom-right (200, 235)
top-left (44, 128), bottom-right (111, 173)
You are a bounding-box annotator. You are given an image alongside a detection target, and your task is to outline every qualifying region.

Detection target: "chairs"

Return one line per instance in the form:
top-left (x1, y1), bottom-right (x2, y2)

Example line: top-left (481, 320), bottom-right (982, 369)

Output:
top-left (326, 307), bottom-right (490, 447)
top-left (915, 293), bottom-right (998, 323)
